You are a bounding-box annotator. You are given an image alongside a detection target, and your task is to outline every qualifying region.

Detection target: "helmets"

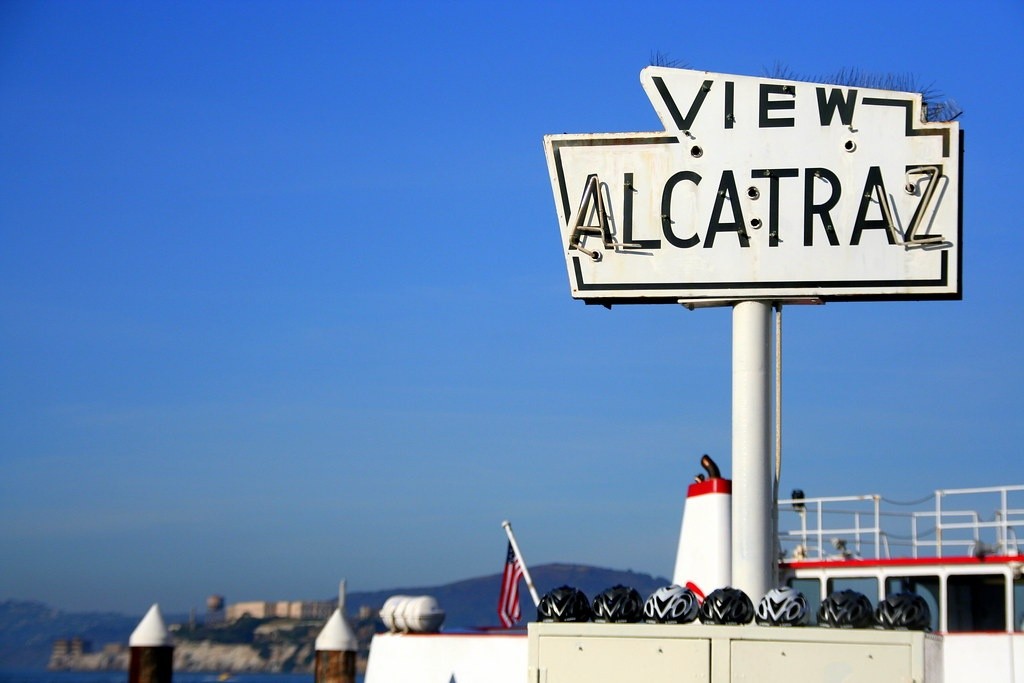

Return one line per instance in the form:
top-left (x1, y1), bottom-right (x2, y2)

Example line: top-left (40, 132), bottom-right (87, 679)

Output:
top-left (642, 586), bottom-right (698, 625)
top-left (816, 589), bottom-right (874, 629)
top-left (755, 586), bottom-right (811, 627)
top-left (700, 588), bottom-right (753, 624)
top-left (536, 584), bottom-right (590, 622)
top-left (874, 592), bottom-right (930, 630)
top-left (592, 584), bottom-right (644, 624)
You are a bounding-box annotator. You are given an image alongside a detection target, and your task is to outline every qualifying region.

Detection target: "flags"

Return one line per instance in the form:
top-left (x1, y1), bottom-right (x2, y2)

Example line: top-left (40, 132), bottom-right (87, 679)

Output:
top-left (497, 540), bottom-right (525, 630)
